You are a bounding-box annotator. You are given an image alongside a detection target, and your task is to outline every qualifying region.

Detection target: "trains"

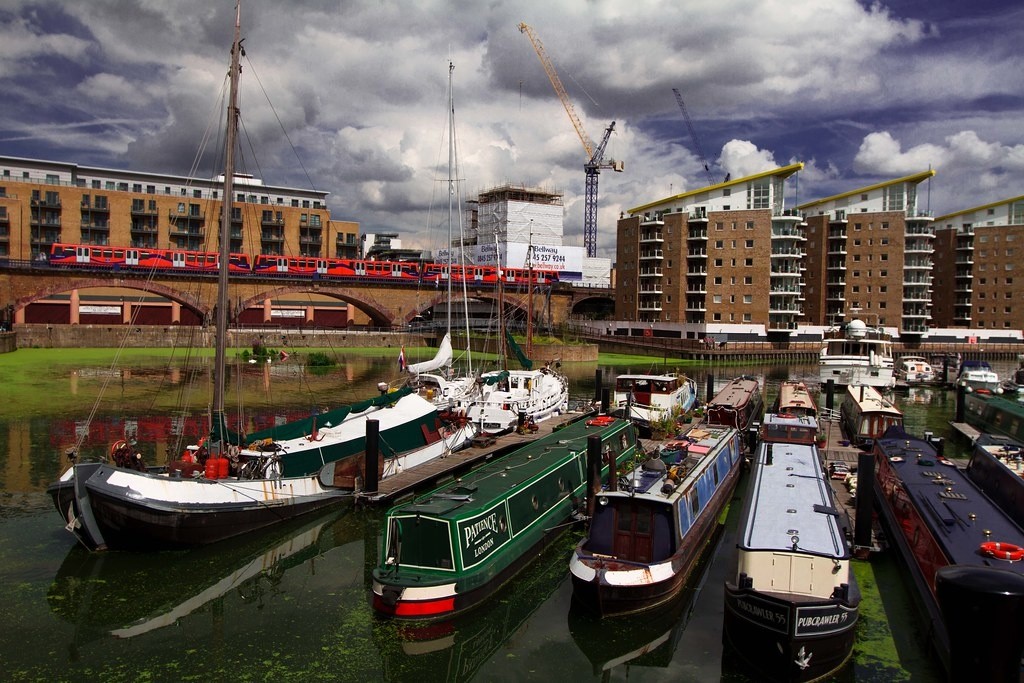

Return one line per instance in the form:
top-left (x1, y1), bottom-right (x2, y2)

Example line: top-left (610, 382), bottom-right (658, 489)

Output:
top-left (50, 241), bottom-right (563, 285)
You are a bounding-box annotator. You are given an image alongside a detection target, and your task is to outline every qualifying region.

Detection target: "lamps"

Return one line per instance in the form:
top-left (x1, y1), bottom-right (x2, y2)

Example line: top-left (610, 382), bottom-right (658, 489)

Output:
top-left (599, 496), bottom-right (609, 505)
top-left (791, 536), bottom-right (799, 552)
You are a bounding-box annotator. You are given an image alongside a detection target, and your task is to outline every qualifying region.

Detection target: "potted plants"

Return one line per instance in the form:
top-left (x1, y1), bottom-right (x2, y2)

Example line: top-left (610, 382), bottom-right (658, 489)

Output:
top-left (816, 434), bottom-right (826, 449)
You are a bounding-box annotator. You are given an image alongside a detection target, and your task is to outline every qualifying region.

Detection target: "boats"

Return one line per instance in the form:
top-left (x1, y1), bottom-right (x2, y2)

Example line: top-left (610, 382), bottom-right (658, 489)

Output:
top-left (963, 433), bottom-right (1024, 531)
top-left (964, 388), bottom-right (1024, 445)
top-left (567, 416), bottom-right (742, 623)
top-left (866, 423), bottom-right (1024, 683)
top-left (368, 415), bottom-right (638, 627)
top-left (703, 373), bottom-right (764, 442)
top-left (772, 379), bottom-right (818, 422)
top-left (893, 355), bottom-right (934, 386)
top-left (720, 441), bottom-right (862, 683)
top-left (607, 360), bottom-right (699, 439)
top-left (818, 317), bottom-right (897, 394)
top-left (955, 360), bottom-right (1002, 394)
top-left (929, 352), bottom-right (962, 385)
top-left (1000, 352), bottom-right (1024, 405)
top-left (840, 379), bottom-right (905, 446)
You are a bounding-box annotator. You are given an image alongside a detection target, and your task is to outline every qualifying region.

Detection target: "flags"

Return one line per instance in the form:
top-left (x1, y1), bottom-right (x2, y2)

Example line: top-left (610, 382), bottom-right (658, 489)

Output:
top-left (398, 346), bottom-right (408, 373)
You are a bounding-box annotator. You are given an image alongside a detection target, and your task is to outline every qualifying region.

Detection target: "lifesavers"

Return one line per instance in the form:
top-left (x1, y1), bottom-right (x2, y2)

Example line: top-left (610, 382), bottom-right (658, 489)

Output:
top-left (388, 388), bottom-right (399, 405)
top-left (979, 541), bottom-right (1024, 561)
top-left (778, 413), bottom-right (796, 418)
top-left (597, 416), bottom-right (614, 422)
top-left (586, 420), bottom-right (607, 426)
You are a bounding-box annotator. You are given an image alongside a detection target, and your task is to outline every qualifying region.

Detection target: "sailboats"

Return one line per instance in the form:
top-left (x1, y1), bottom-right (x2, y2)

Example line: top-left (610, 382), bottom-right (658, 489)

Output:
top-left (371, 58), bottom-right (571, 442)
top-left (42, 0), bottom-right (477, 558)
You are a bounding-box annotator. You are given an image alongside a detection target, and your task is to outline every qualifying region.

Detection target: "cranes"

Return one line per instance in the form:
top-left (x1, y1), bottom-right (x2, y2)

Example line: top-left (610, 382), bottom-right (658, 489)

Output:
top-left (672, 87), bottom-right (716, 186)
top-left (516, 20), bottom-right (626, 257)
